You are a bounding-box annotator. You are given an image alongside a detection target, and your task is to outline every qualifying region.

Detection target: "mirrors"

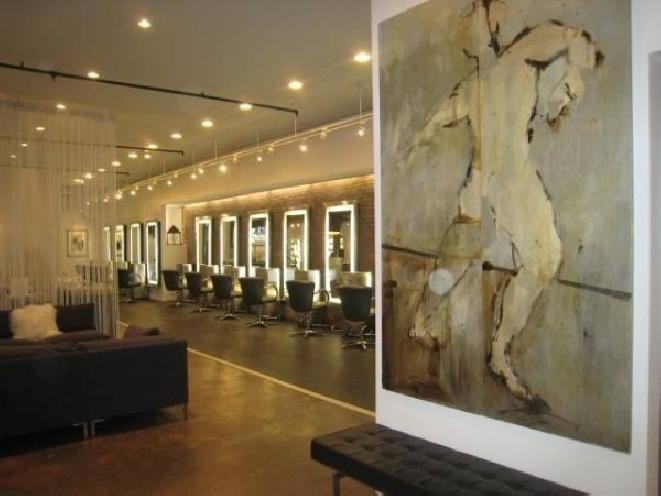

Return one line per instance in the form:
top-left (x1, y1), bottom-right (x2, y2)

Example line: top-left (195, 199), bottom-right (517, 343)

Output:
top-left (102, 202), bottom-right (355, 286)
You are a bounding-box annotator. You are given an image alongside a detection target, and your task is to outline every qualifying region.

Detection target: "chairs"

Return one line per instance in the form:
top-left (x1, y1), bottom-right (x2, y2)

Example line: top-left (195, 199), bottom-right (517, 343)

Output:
top-left (162, 270), bottom-right (373, 348)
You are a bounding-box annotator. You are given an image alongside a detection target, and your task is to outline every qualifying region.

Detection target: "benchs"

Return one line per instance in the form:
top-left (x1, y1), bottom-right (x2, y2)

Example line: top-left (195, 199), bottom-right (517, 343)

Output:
top-left (311, 422), bottom-right (592, 495)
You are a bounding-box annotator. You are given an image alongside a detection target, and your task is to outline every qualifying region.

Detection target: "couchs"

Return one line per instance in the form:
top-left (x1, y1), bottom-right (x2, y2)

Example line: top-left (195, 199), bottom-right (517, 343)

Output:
top-left (1, 299), bottom-right (188, 454)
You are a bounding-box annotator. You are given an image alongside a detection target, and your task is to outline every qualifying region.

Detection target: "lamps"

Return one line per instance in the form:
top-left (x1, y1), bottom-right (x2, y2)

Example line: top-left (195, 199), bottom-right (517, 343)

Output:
top-left (111, 87), bottom-right (365, 202)
